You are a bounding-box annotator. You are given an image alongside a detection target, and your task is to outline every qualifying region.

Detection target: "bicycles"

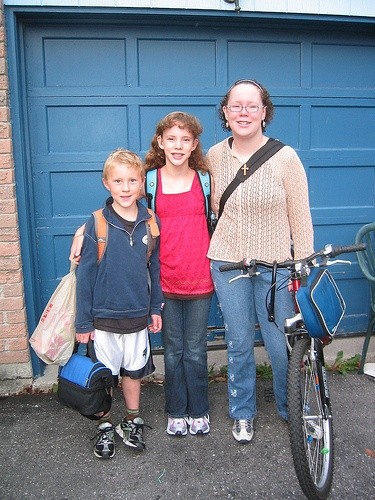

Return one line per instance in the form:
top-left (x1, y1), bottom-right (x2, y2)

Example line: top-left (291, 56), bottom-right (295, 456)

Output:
top-left (219, 241), bottom-right (368, 500)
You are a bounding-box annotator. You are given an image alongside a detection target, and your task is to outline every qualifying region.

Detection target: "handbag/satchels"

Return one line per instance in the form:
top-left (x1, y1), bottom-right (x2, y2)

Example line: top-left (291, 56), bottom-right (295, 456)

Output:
top-left (294, 268), bottom-right (346, 337)
top-left (29, 259), bottom-right (76, 366)
top-left (57, 338), bottom-right (113, 415)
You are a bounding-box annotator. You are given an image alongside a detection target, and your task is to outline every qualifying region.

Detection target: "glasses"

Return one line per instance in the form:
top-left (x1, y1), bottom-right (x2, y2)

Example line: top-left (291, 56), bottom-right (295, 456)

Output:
top-left (226, 104), bottom-right (264, 113)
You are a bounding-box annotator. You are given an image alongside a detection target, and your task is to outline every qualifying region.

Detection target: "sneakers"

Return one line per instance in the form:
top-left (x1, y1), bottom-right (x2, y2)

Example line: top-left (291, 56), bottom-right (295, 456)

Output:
top-left (306, 420), bottom-right (322, 439)
top-left (189, 414), bottom-right (210, 435)
top-left (233, 416), bottom-right (255, 443)
top-left (115, 415), bottom-right (153, 451)
top-left (89, 423), bottom-right (115, 459)
top-left (167, 418), bottom-right (187, 436)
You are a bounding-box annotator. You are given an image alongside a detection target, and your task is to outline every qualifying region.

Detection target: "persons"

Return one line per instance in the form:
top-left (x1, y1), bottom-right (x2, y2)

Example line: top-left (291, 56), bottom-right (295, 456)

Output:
top-left (76, 149), bottom-right (165, 458)
top-left (69, 112), bottom-right (217, 434)
top-left (202, 79), bottom-right (314, 442)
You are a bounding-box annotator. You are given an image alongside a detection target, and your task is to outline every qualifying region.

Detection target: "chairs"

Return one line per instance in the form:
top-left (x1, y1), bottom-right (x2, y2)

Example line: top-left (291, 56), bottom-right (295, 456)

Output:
top-left (355, 222), bottom-right (375, 374)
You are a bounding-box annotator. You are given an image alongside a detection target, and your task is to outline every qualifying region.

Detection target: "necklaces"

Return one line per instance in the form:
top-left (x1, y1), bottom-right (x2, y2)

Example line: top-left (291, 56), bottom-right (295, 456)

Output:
top-left (241, 163), bottom-right (249, 175)
